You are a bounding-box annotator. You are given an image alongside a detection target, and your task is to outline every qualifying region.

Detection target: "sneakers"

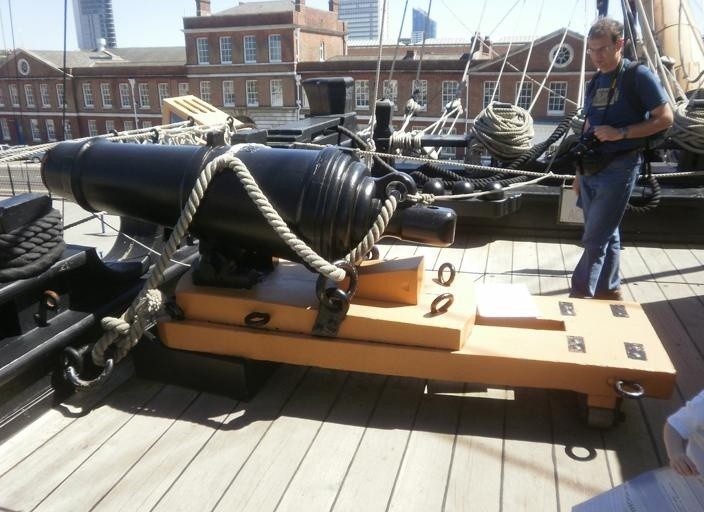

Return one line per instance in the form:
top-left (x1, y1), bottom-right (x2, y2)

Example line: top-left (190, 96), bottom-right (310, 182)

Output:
top-left (591, 286), bottom-right (625, 301)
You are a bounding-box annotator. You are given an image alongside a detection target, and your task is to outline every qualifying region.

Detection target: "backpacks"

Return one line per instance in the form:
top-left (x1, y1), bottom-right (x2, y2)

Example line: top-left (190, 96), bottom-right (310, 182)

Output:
top-left (620, 60), bottom-right (673, 151)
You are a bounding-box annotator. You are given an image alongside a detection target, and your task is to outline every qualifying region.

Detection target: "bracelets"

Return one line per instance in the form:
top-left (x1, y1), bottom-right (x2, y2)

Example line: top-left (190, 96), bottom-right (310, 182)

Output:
top-left (620, 126), bottom-right (629, 139)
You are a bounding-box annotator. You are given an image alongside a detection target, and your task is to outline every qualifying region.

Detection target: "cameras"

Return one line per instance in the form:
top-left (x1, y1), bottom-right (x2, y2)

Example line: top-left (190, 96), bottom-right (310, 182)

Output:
top-left (571, 133), bottom-right (599, 157)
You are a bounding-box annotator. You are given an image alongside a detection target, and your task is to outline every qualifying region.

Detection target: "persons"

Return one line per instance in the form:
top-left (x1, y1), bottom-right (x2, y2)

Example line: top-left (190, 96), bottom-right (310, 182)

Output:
top-left (569, 20), bottom-right (673, 301)
top-left (663, 390), bottom-right (704, 476)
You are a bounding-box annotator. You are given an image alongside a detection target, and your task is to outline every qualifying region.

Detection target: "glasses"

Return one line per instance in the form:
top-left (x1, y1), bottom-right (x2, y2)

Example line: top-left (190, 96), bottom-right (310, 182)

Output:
top-left (586, 43), bottom-right (614, 57)
